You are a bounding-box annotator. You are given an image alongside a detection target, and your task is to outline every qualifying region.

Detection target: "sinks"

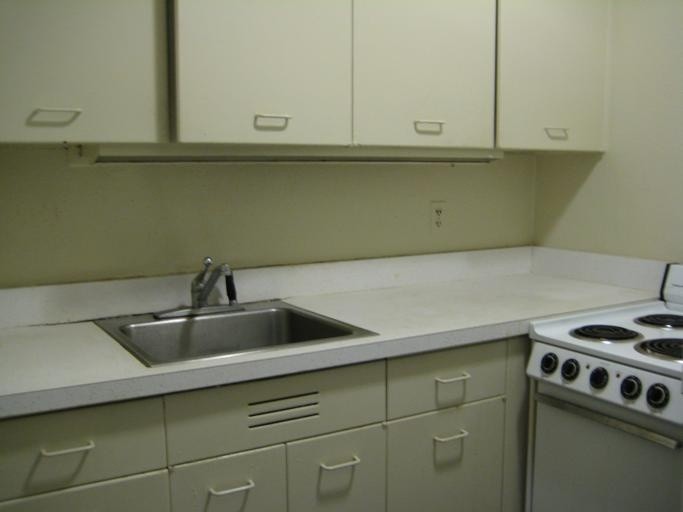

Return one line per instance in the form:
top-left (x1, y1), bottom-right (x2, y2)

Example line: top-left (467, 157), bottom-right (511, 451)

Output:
top-left (94, 299), bottom-right (382, 369)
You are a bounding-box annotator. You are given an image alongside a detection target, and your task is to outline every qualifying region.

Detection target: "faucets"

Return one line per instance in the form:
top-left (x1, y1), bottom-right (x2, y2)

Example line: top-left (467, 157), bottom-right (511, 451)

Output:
top-left (191, 261), bottom-right (237, 309)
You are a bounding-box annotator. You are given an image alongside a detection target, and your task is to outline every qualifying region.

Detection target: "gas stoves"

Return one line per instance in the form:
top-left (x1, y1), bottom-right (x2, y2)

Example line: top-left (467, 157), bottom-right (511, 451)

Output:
top-left (523, 300), bottom-right (682, 427)
